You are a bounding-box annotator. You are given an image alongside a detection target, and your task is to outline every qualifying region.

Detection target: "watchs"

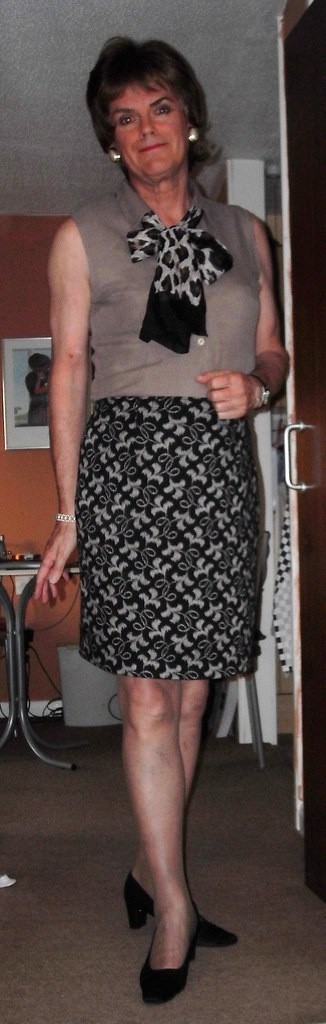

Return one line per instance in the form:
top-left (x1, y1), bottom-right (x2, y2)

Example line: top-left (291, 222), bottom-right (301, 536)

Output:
top-left (247, 372), bottom-right (271, 410)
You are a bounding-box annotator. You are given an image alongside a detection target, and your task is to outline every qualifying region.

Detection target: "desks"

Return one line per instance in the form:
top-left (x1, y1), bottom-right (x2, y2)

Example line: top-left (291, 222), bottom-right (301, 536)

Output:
top-left (0, 568), bottom-right (92, 771)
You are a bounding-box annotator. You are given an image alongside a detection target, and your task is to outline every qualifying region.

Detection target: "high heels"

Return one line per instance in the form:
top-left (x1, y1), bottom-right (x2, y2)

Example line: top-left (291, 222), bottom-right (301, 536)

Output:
top-left (123, 871), bottom-right (239, 947)
top-left (139, 900), bottom-right (200, 1004)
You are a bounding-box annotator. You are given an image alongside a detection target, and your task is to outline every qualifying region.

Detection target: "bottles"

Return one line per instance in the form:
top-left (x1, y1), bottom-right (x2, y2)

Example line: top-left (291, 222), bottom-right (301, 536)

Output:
top-left (0, 535), bottom-right (7, 559)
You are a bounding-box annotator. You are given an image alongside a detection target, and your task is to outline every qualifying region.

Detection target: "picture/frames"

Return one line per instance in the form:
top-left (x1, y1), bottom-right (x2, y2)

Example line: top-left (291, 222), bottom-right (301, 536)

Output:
top-left (1, 337), bottom-right (51, 450)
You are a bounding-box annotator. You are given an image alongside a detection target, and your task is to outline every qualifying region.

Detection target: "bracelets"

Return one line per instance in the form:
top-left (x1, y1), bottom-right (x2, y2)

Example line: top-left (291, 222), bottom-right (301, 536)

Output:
top-left (55, 513), bottom-right (77, 523)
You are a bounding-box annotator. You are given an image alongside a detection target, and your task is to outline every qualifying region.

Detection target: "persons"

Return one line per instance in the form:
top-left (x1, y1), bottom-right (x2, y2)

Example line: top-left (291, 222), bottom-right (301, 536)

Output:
top-left (25, 353), bottom-right (53, 426)
top-left (34, 38), bottom-right (290, 1007)
